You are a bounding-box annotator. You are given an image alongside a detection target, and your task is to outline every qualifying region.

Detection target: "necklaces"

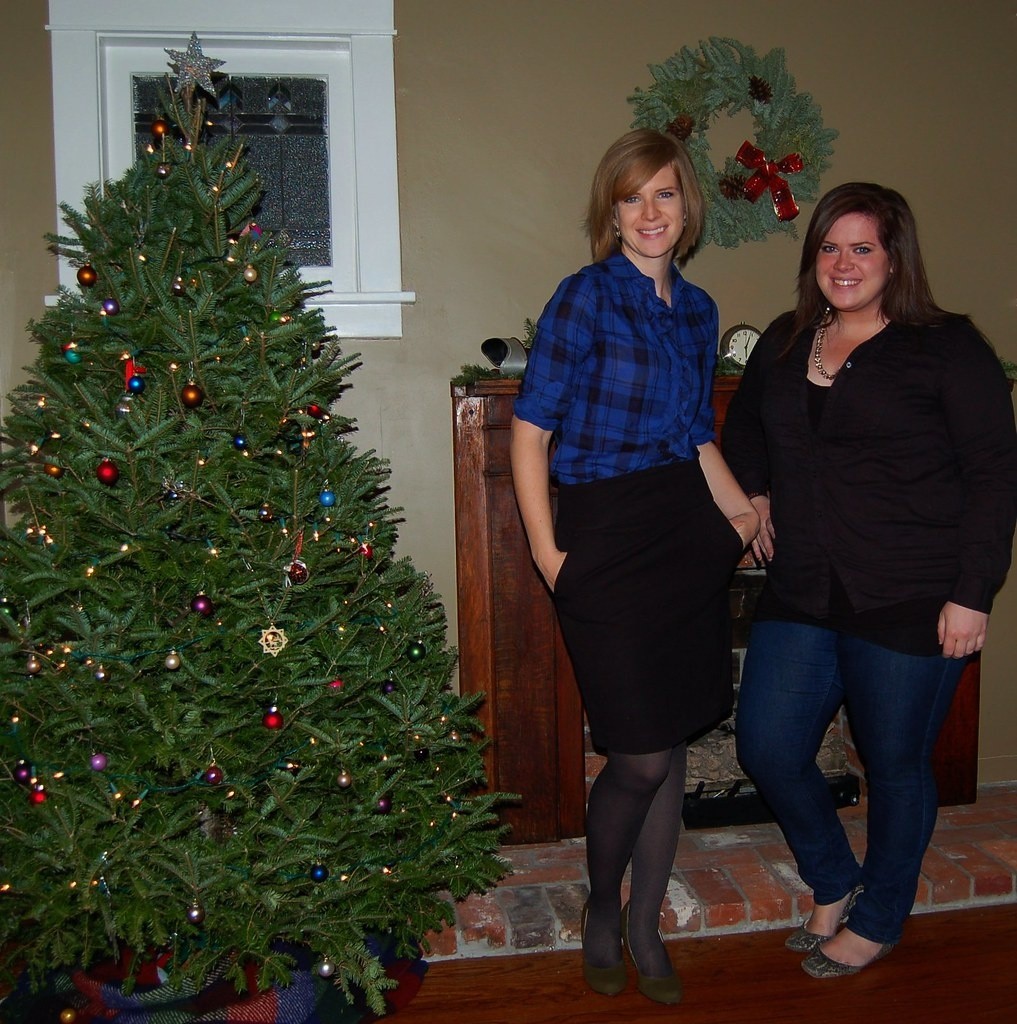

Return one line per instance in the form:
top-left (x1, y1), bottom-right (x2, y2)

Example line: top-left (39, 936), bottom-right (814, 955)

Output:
top-left (814, 307), bottom-right (835, 380)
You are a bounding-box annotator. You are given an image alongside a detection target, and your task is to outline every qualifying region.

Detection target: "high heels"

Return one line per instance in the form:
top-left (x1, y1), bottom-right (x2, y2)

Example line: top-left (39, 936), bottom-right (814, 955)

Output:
top-left (783, 880), bottom-right (868, 952)
top-left (618, 902), bottom-right (683, 1006)
top-left (580, 898), bottom-right (626, 997)
top-left (800, 928), bottom-right (897, 978)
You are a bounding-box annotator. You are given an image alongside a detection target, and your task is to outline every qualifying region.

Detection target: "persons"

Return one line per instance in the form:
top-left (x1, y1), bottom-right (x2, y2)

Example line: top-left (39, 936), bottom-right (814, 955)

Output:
top-left (719, 181), bottom-right (1017, 978)
top-left (509, 128), bottom-right (761, 1006)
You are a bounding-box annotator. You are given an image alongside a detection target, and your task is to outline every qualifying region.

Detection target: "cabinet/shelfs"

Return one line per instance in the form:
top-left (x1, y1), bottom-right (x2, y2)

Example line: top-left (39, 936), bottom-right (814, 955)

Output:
top-left (454, 357), bottom-right (1017, 846)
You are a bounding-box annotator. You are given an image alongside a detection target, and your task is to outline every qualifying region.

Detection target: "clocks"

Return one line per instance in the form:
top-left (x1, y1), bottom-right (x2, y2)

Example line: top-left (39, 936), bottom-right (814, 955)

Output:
top-left (720, 322), bottom-right (764, 369)
